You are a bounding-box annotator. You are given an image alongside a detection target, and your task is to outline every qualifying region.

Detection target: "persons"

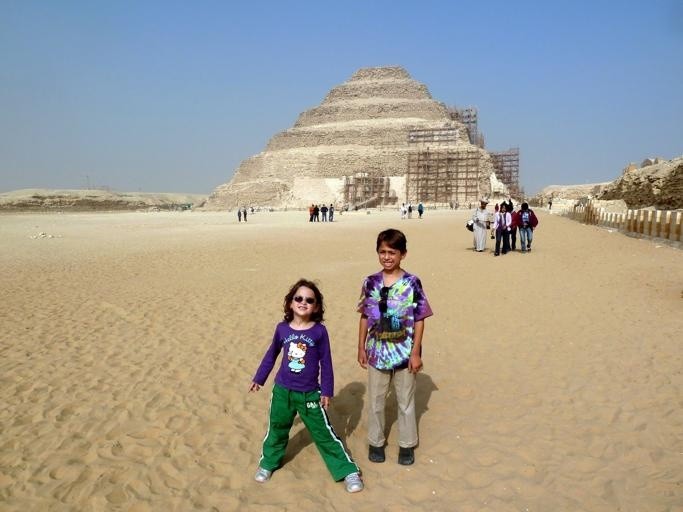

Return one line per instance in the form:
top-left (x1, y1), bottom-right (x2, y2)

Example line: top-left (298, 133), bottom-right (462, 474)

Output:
top-left (245, 279), bottom-right (363, 494)
top-left (237, 209), bottom-right (240, 221)
top-left (407, 201), bottom-right (412, 219)
top-left (243, 209), bottom-right (247, 221)
top-left (356, 228), bottom-right (432, 466)
top-left (547, 197), bottom-right (552, 210)
top-left (465, 198), bottom-right (538, 256)
top-left (417, 202), bottom-right (423, 218)
top-left (399, 202), bottom-right (406, 219)
top-left (308, 203), bottom-right (333, 222)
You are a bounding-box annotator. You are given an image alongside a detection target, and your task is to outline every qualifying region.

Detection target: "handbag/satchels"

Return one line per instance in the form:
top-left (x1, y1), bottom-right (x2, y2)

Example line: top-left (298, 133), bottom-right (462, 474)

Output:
top-left (466, 222), bottom-right (473, 231)
top-left (489, 234), bottom-right (495, 239)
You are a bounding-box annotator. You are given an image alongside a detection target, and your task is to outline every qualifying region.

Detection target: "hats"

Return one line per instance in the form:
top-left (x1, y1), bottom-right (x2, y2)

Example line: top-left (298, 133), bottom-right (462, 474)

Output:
top-left (479, 198), bottom-right (488, 204)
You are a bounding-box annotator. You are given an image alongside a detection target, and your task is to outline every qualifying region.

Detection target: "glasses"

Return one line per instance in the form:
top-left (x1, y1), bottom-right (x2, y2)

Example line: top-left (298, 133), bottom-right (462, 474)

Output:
top-left (291, 296), bottom-right (317, 304)
top-left (378, 286), bottom-right (389, 314)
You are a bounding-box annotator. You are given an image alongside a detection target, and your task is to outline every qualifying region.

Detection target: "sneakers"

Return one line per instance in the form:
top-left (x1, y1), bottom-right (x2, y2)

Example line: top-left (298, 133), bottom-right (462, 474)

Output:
top-left (495, 245), bottom-right (531, 255)
top-left (344, 471), bottom-right (363, 493)
top-left (398, 447), bottom-right (414, 465)
top-left (255, 466), bottom-right (272, 483)
top-left (368, 444), bottom-right (385, 463)
